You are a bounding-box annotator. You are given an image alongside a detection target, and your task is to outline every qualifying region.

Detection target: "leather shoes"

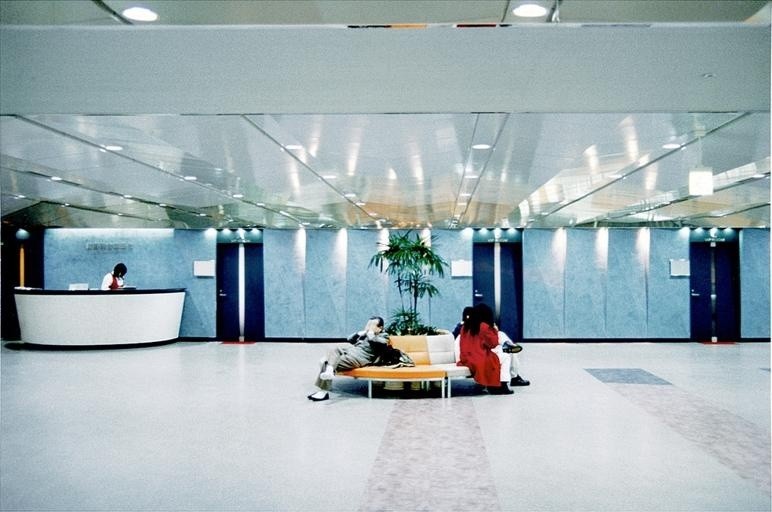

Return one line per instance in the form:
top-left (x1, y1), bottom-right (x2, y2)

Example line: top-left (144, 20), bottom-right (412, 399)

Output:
top-left (510, 374), bottom-right (530, 386)
top-left (502, 342), bottom-right (522, 353)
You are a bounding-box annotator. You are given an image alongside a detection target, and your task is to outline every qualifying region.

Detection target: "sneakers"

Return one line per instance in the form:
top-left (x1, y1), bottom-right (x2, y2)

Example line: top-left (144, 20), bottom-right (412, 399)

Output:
top-left (307, 391), bottom-right (330, 401)
top-left (320, 372), bottom-right (335, 381)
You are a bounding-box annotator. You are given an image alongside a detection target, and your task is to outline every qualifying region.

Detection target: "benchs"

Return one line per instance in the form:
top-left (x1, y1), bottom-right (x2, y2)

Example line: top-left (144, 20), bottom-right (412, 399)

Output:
top-left (336, 328), bottom-right (472, 399)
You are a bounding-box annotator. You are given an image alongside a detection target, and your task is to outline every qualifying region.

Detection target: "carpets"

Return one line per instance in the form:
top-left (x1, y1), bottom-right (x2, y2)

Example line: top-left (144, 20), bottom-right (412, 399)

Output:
top-left (703, 342), bottom-right (735, 344)
top-left (222, 341), bottom-right (256, 344)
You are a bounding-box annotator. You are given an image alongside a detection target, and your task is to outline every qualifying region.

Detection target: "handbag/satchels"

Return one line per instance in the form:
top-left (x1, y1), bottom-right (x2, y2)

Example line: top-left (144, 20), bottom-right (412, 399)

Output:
top-left (379, 344), bottom-right (400, 364)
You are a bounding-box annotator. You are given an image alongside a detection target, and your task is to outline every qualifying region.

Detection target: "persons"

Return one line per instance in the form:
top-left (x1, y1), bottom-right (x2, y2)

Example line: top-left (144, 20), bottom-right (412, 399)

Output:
top-left (308, 316), bottom-right (389, 401)
top-left (452, 306), bottom-right (530, 385)
top-left (101, 262), bottom-right (128, 290)
top-left (455, 303), bottom-right (514, 394)
